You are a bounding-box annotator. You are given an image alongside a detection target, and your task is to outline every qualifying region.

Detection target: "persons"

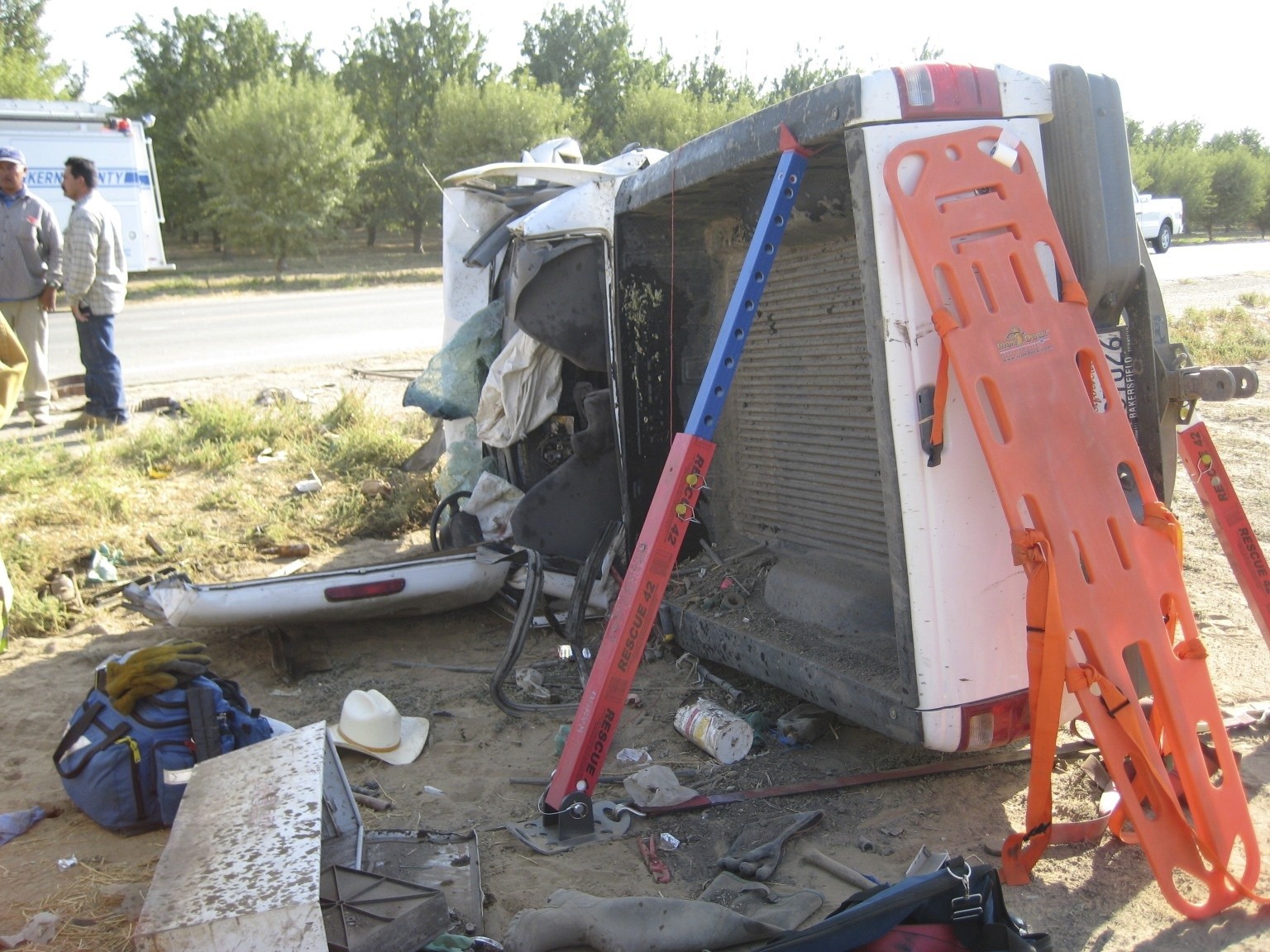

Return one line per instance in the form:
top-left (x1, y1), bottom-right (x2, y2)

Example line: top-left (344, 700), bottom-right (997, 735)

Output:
top-left (61, 157), bottom-right (129, 430)
top-left (0, 147), bottom-right (63, 427)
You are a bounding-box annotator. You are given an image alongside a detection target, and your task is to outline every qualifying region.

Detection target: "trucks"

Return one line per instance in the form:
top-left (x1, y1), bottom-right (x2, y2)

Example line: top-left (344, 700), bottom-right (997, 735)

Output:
top-left (438, 58), bottom-right (1260, 761)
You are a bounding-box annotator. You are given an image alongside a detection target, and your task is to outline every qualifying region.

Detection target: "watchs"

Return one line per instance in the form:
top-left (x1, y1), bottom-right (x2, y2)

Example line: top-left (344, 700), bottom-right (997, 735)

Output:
top-left (46, 281), bottom-right (59, 289)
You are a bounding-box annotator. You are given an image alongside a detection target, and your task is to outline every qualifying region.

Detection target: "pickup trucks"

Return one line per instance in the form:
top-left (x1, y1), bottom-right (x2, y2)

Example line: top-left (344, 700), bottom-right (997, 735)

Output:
top-left (1131, 183), bottom-right (1184, 254)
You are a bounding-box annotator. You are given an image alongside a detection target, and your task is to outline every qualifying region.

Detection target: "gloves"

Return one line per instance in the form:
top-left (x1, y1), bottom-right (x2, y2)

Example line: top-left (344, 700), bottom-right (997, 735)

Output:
top-left (109, 665), bottom-right (208, 716)
top-left (107, 637), bottom-right (212, 690)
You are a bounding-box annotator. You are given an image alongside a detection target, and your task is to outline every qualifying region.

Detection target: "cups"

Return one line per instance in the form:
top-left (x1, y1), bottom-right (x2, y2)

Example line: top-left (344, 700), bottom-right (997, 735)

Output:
top-left (671, 692), bottom-right (754, 765)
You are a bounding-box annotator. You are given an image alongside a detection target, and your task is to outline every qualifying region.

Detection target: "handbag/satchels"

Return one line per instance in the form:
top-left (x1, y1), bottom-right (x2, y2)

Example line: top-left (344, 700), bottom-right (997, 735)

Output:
top-left (753, 855), bottom-right (1053, 952)
top-left (53, 653), bottom-right (273, 837)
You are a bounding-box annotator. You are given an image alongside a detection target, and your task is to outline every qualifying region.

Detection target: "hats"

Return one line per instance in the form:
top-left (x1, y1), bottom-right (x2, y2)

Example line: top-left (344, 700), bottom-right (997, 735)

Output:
top-left (329, 689), bottom-right (430, 765)
top-left (0, 146), bottom-right (26, 166)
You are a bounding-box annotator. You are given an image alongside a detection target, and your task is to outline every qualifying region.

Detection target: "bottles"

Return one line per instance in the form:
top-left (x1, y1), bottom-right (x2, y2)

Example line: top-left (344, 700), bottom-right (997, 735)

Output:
top-left (261, 544), bottom-right (309, 556)
top-left (554, 724), bottom-right (571, 755)
top-left (778, 703), bottom-right (832, 745)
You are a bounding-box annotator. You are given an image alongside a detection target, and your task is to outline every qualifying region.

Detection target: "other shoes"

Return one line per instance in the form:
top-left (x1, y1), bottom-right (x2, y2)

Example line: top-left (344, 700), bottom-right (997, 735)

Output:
top-left (64, 411), bottom-right (113, 429)
top-left (32, 413), bottom-right (50, 427)
